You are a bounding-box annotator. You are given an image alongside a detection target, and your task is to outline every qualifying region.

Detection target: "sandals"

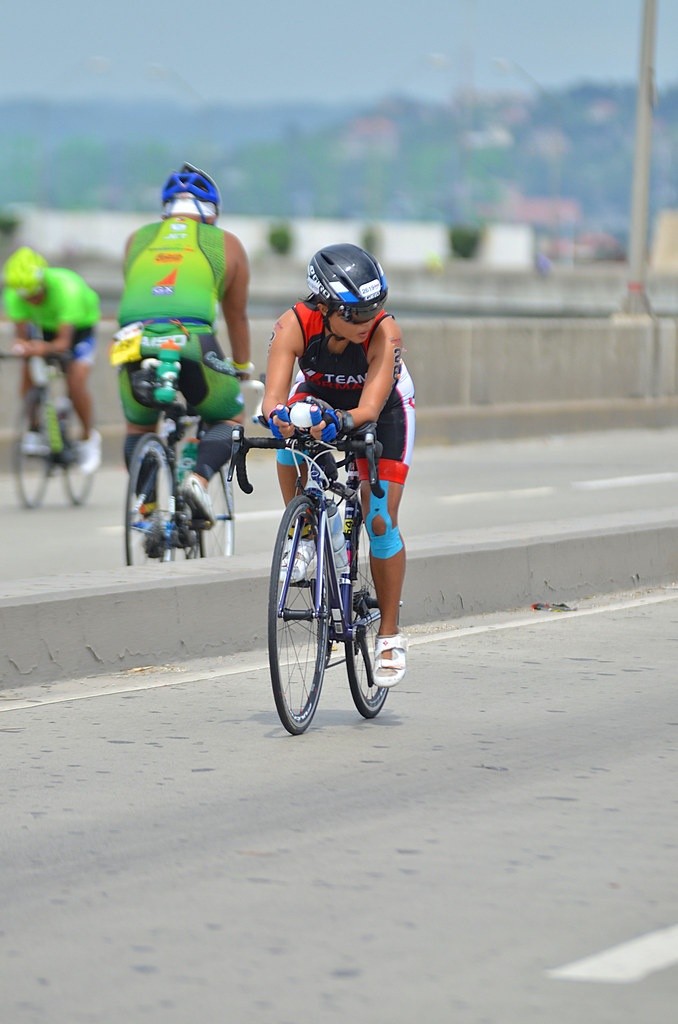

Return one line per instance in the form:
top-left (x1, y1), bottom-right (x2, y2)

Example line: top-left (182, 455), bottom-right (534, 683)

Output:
top-left (279, 539), bottom-right (316, 583)
top-left (372, 626), bottom-right (408, 688)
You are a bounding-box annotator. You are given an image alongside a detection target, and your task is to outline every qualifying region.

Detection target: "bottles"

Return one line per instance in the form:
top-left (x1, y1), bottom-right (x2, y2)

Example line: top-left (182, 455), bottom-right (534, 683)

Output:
top-left (325, 499), bottom-right (348, 568)
top-left (178, 436), bottom-right (200, 483)
top-left (154, 339), bottom-right (180, 402)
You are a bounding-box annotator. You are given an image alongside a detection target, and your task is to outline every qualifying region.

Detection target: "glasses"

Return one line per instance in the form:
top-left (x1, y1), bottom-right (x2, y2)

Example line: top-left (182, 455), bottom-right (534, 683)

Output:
top-left (337, 296), bottom-right (387, 324)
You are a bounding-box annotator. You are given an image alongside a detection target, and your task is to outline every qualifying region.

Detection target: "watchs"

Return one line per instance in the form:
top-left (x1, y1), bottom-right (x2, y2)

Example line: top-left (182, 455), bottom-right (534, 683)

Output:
top-left (338, 410), bottom-right (354, 434)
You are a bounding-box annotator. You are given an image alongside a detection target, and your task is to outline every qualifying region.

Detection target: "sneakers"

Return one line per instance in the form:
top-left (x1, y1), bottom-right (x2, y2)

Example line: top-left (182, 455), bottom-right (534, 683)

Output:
top-left (181, 476), bottom-right (215, 526)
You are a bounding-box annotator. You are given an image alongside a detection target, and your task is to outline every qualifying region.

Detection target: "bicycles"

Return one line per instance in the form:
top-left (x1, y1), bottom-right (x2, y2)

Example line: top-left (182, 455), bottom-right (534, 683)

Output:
top-left (124, 374), bottom-right (270, 566)
top-left (0, 351), bottom-right (94, 510)
top-left (226, 403), bottom-right (403, 735)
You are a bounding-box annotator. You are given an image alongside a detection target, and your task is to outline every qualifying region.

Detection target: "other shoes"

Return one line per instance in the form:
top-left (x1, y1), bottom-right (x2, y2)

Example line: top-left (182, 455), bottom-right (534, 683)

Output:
top-left (19, 432), bottom-right (50, 457)
top-left (80, 431), bottom-right (103, 473)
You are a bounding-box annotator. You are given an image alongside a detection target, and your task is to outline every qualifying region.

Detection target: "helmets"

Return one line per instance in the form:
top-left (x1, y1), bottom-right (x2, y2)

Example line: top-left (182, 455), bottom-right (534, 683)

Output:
top-left (306, 243), bottom-right (388, 307)
top-left (161, 161), bottom-right (222, 217)
top-left (4, 247), bottom-right (48, 299)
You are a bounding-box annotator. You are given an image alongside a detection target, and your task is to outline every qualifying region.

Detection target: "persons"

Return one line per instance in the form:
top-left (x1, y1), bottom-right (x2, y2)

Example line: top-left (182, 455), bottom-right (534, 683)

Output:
top-left (108, 162), bottom-right (251, 533)
top-left (262, 243), bottom-right (415, 688)
top-left (1, 246), bottom-right (103, 476)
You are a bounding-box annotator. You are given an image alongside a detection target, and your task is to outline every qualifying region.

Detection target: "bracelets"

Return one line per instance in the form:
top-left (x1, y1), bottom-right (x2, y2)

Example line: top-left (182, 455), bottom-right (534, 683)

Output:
top-left (231, 358), bottom-right (249, 370)
